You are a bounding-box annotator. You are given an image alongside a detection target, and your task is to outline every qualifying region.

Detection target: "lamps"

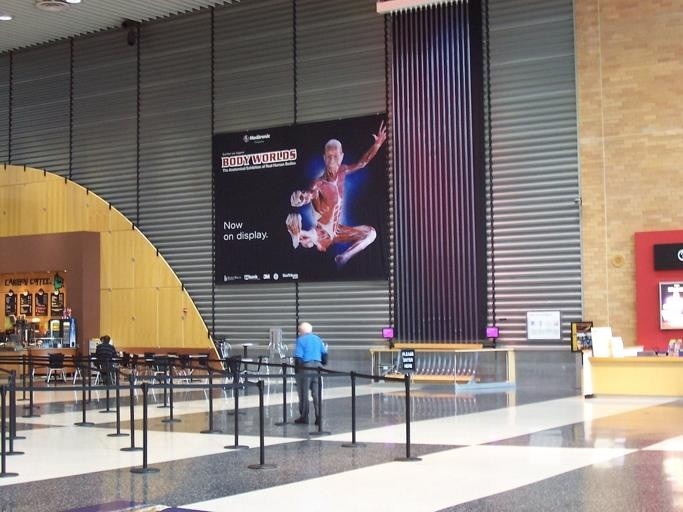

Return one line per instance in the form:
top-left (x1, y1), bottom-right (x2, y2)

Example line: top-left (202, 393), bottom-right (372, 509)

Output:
top-left (53, 271), bottom-right (59, 296)
top-left (23, 272), bottom-right (29, 297)
top-left (381, 328), bottom-right (395, 349)
top-left (8, 272), bottom-right (14, 297)
top-left (37, 272), bottom-right (44, 297)
top-left (486, 327), bottom-right (499, 346)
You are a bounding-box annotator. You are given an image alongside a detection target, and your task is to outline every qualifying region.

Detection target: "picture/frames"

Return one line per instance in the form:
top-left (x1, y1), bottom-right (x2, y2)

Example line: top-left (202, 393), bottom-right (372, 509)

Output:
top-left (51, 293), bottom-right (65, 316)
top-left (20, 294), bottom-right (32, 316)
top-left (35, 293), bottom-right (48, 316)
top-left (5, 294), bottom-right (17, 316)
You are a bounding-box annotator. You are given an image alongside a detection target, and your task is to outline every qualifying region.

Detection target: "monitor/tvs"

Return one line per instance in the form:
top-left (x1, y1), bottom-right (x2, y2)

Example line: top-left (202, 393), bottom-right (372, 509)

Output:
top-left (382, 328), bottom-right (395, 339)
top-left (486, 327), bottom-right (497, 339)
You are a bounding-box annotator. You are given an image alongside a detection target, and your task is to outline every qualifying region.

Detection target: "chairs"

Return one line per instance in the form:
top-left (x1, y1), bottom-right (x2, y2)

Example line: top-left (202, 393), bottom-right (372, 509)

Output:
top-left (46, 352), bottom-right (270, 402)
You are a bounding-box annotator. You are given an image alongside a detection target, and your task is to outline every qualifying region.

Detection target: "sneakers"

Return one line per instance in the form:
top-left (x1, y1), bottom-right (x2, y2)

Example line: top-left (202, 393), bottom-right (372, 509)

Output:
top-left (294, 417), bottom-right (322, 425)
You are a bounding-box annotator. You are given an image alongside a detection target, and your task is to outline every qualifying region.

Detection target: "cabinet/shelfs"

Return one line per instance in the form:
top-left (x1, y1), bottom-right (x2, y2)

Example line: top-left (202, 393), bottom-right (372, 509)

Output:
top-left (48, 319), bottom-right (76, 347)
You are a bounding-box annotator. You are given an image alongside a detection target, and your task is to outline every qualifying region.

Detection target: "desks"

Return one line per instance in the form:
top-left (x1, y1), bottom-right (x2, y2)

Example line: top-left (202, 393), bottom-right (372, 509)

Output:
top-left (370, 347), bottom-right (401, 383)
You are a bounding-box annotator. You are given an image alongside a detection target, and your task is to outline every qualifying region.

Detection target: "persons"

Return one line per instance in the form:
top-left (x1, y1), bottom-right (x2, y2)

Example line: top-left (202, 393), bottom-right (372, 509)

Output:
top-left (280, 117), bottom-right (386, 266)
top-left (94, 334), bottom-right (117, 384)
top-left (95, 336), bottom-right (104, 354)
top-left (290, 320), bottom-right (329, 425)
